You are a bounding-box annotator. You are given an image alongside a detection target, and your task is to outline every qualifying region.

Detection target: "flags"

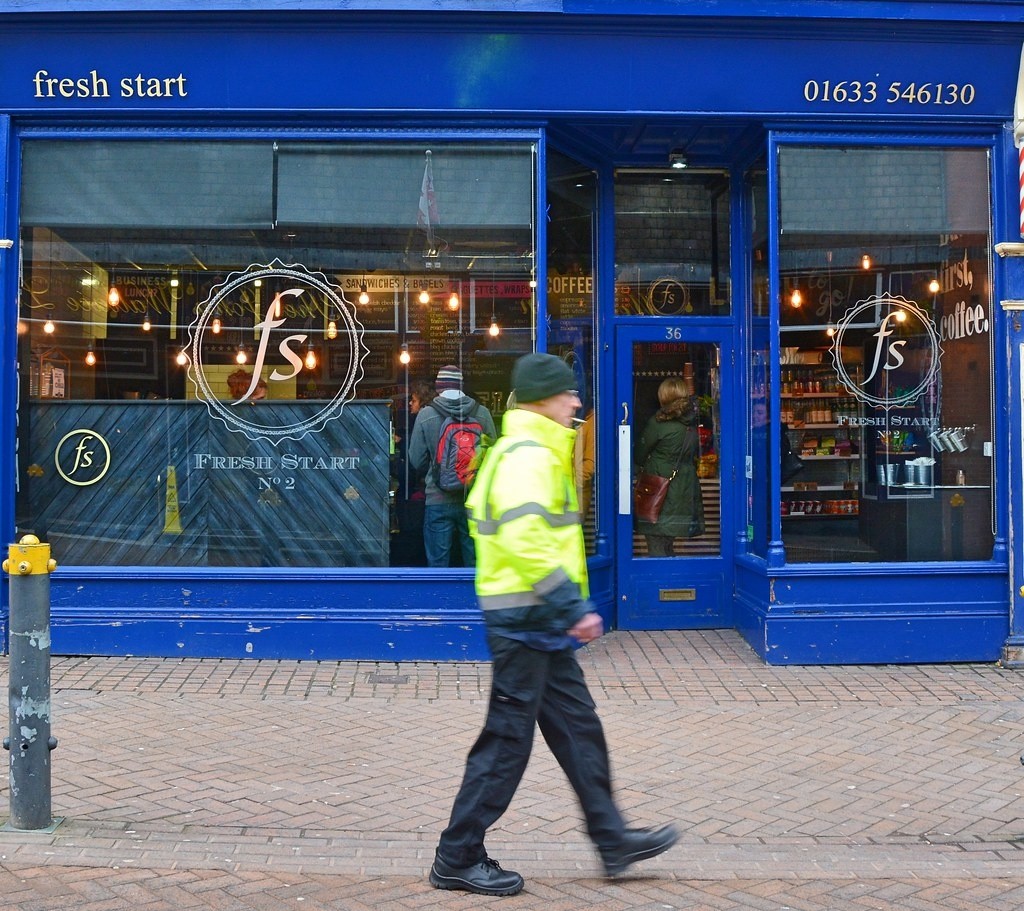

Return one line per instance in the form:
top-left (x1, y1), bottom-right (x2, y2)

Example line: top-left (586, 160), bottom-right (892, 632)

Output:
top-left (416, 155), bottom-right (440, 229)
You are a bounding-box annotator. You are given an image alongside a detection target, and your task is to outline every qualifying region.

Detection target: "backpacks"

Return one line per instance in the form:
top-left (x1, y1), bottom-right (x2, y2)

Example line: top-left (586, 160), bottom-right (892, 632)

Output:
top-left (428, 401), bottom-right (486, 491)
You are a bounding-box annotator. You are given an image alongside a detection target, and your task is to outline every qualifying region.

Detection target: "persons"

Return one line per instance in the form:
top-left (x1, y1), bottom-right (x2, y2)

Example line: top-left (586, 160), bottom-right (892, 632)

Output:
top-left (429, 352), bottom-right (680, 897)
top-left (752, 398), bottom-right (791, 538)
top-left (227, 370), bottom-right (268, 400)
top-left (634, 378), bottom-right (705, 557)
top-left (390, 364), bottom-right (498, 567)
top-left (570, 393), bottom-right (596, 525)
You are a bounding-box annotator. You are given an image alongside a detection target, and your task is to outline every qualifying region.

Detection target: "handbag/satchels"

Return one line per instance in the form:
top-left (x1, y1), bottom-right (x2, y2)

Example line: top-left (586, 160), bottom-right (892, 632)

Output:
top-left (633, 472), bottom-right (670, 524)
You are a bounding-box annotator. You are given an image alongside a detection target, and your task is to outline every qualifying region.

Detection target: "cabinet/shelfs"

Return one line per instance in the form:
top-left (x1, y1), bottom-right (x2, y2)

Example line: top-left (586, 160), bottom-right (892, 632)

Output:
top-left (777, 344), bottom-right (940, 520)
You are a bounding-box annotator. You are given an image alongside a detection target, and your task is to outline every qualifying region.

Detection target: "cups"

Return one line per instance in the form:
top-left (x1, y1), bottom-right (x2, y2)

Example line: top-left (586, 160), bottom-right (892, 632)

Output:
top-left (927, 427), bottom-right (970, 453)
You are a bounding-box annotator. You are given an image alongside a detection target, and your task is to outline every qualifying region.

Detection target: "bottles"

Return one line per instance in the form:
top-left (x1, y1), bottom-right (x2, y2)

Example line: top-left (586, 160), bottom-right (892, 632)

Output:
top-left (873, 382), bottom-right (921, 452)
top-left (955, 469), bottom-right (965, 485)
top-left (767, 367), bottom-right (860, 425)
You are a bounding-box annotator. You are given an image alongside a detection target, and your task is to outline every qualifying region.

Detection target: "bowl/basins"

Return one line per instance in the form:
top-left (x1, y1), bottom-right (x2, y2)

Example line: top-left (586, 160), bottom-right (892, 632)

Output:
top-left (876, 462), bottom-right (931, 486)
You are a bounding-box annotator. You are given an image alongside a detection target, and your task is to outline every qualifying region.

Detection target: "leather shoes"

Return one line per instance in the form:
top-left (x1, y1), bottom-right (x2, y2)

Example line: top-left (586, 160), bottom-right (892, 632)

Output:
top-left (600, 825), bottom-right (680, 875)
top-left (428, 850), bottom-right (524, 896)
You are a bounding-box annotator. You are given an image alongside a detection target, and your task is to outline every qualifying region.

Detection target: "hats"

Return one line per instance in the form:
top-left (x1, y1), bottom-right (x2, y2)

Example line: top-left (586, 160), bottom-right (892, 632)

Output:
top-left (513, 352), bottom-right (578, 403)
top-left (435, 365), bottom-right (463, 392)
